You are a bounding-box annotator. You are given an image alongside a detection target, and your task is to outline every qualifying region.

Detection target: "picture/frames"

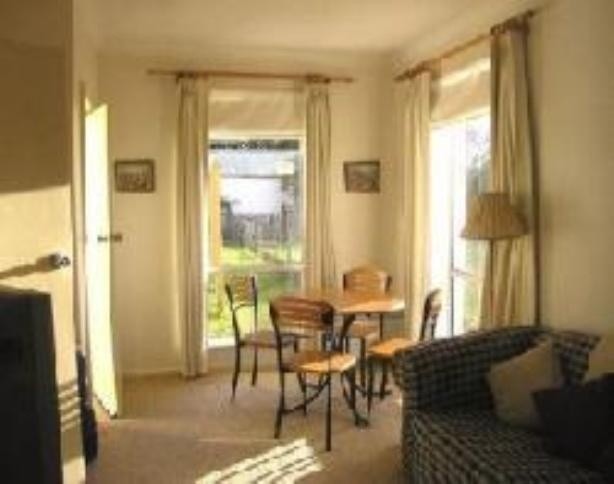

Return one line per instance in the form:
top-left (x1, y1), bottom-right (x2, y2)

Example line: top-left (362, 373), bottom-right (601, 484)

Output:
top-left (343, 160), bottom-right (381, 193)
top-left (115, 159), bottom-right (156, 193)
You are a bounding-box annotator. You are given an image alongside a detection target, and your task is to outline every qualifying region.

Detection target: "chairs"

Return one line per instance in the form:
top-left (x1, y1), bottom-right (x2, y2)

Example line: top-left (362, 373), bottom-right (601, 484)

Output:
top-left (368, 288), bottom-right (443, 411)
top-left (336, 268), bottom-right (392, 398)
top-left (269, 294), bottom-right (368, 452)
top-left (225, 274), bottom-right (301, 396)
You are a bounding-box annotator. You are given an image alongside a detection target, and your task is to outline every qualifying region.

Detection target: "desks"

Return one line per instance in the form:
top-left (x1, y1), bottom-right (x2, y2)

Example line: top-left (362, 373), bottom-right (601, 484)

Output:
top-left (280, 293), bottom-right (404, 428)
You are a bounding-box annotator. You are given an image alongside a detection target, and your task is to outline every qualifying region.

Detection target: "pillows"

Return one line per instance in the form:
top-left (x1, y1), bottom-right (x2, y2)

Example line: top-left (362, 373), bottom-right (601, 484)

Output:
top-left (489, 334), bottom-right (614, 459)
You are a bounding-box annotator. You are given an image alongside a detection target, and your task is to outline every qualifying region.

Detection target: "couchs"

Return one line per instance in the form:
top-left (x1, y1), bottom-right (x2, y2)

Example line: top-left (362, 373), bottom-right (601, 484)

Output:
top-left (392, 325), bottom-right (614, 484)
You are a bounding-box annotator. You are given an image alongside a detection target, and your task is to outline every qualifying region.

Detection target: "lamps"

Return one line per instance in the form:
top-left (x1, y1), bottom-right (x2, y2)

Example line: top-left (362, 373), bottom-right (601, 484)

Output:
top-left (459, 193), bottom-right (530, 328)
